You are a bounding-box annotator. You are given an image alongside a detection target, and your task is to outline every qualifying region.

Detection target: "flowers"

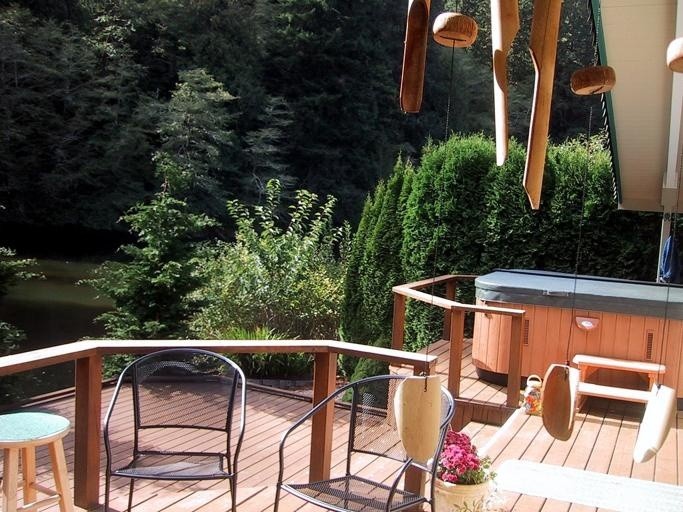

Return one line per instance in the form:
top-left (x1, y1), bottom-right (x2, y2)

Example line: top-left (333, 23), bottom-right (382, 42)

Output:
top-left (434, 429), bottom-right (499, 485)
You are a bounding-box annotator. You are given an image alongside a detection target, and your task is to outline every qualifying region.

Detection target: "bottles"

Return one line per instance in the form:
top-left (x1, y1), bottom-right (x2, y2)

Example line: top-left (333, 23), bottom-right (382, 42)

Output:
top-left (524, 374), bottom-right (542, 416)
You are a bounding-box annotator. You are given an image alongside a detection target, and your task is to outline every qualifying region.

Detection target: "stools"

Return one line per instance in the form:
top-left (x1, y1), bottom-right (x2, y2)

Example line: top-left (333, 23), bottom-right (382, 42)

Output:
top-left (0, 411), bottom-right (75, 512)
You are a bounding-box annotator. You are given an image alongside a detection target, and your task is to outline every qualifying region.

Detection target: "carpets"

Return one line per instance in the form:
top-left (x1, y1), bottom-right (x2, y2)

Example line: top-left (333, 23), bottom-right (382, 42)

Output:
top-left (489, 457), bottom-right (683, 512)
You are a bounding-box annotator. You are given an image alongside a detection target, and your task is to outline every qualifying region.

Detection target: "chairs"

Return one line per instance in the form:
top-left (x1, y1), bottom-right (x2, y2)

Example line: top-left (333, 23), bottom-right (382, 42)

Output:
top-left (274, 374), bottom-right (457, 512)
top-left (103, 347), bottom-right (247, 512)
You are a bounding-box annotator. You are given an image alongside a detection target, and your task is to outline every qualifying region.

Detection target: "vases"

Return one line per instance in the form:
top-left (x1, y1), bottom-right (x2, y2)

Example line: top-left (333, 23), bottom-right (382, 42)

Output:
top-left (430, 473), bottom-right (499, 512)
